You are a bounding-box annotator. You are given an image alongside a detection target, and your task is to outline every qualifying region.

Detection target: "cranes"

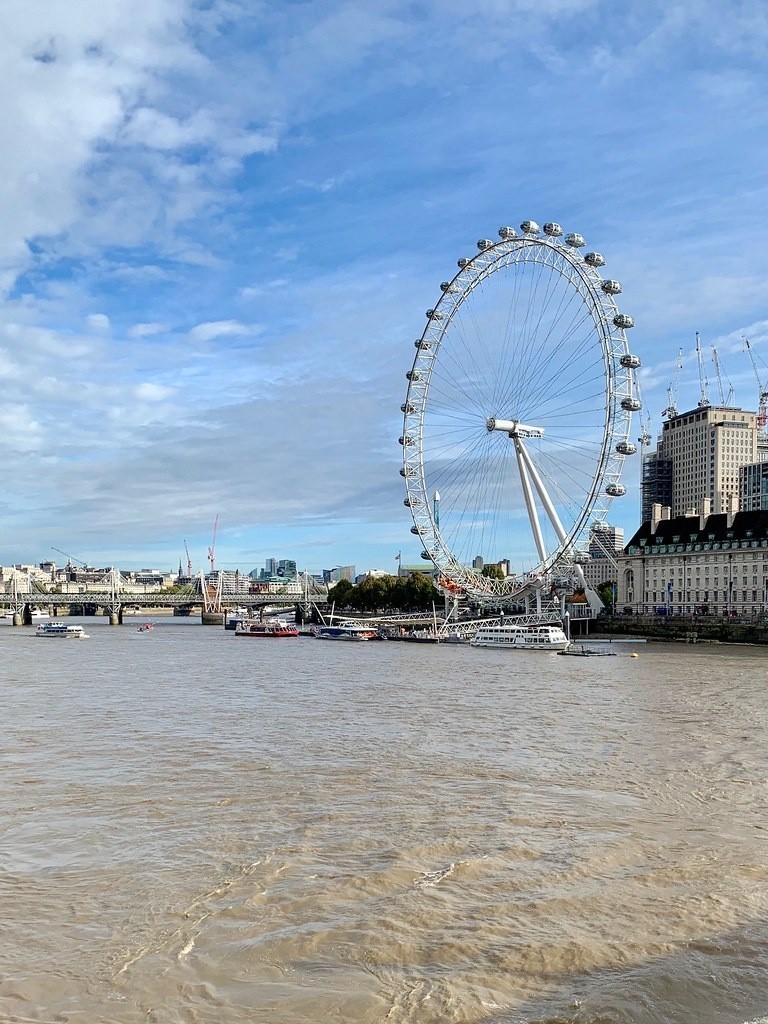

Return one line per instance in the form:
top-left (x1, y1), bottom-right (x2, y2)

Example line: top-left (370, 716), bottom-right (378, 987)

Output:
top-left (184, 539), bottom-right (192, 577)
top-left (662, 330), bottom-right (768, 419)
top-left (206, 513), bottom-right (220, 570)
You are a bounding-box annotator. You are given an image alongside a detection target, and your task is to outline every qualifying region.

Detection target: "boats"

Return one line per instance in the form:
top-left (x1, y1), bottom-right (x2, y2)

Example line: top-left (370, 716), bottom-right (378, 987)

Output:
top-left (4, 606), bottom-right (50, 619)
top-left (316, 603), bottom-right (380, 643)
top-left (223, 608), bottom-right (288, 631)
top-left (234, 608), bottom-right (299, 637)
top-left (469, 623), bottom-right (570, 651)
top-left (35, 622), bottom-right (87, 640)
